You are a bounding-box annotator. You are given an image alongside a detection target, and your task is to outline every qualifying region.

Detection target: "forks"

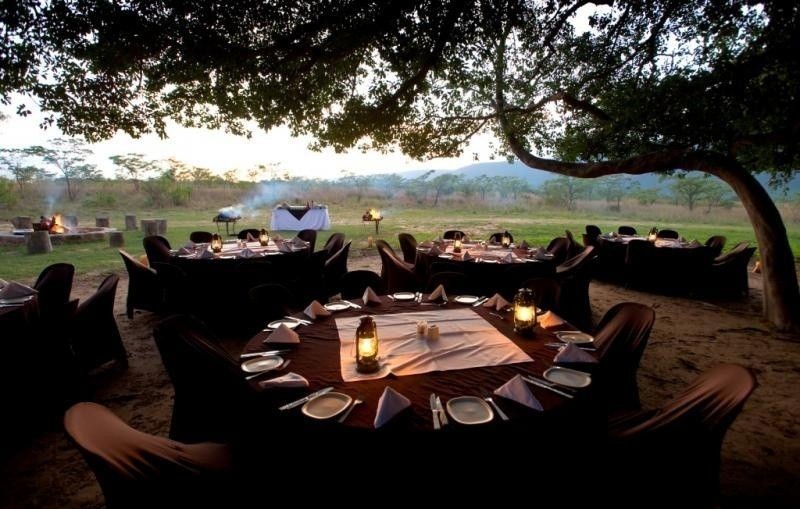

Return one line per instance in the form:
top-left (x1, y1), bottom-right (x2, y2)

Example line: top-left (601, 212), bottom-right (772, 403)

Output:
top-left (481, 391), bottom-right (509, 421)
top-left (339, 392), bottom-right (363, 423)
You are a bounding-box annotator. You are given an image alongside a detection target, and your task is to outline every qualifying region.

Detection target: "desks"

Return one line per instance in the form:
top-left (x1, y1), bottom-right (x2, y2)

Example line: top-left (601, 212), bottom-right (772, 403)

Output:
top-left (271, 205), bottom-right (330, 231)
top-left (213, 217), bottom-right (241, 236)
top-left (362, 216), bottom-right (383, 235)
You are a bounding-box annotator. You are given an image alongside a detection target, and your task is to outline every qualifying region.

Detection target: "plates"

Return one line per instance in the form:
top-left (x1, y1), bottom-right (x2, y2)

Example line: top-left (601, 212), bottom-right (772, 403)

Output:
top-left (543, 365), bottom-right (592, 388)
top-left (556, 331), bottom-right (595, 345)
top-left (323, 300), bottom-right (352, 312)
top-left (301, 393), bottom-right (353, 420)
top-left (446, 396), bottom-right (495, 426)
top-left (416, 239), bottom-right (554, 264)
top-left (454, 294), bottom-right (479, 305)
top-left (0, 295), bottom-right (34, 304)
top-left (266, 319), bottom-right (299, 330)
top-left (240, 354), bottom-right (285, 374)
top-left (392, 291), bottom-right (415, 300)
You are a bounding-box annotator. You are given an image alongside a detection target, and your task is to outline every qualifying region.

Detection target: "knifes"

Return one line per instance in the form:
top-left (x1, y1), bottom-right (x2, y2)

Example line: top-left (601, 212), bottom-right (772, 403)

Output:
top-left (436, 397), bottom-right (448, 426)
top-left (521, 374), bottom-right (575, 400)
top-left (281, 384), bottom-right (332, 413)
top-left (428, 390), bottom-right (441, 431)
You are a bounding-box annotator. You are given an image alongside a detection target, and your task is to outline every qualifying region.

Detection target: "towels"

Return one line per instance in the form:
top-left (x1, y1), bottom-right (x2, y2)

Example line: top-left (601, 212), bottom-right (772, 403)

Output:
top-left (0, 281), bottom-right (38, 299)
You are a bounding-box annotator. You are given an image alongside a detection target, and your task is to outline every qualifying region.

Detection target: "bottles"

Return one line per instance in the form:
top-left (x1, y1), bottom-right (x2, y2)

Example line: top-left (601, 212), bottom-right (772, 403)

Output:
top-left (236, 238), bottom-right (249, 248)
top-left (416, 320), bottom-right (440, 341)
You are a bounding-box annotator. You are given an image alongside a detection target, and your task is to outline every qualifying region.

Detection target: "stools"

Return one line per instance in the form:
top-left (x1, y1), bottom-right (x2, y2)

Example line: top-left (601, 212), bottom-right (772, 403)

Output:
top-left (10, 214), bottom-right (168, 255)
